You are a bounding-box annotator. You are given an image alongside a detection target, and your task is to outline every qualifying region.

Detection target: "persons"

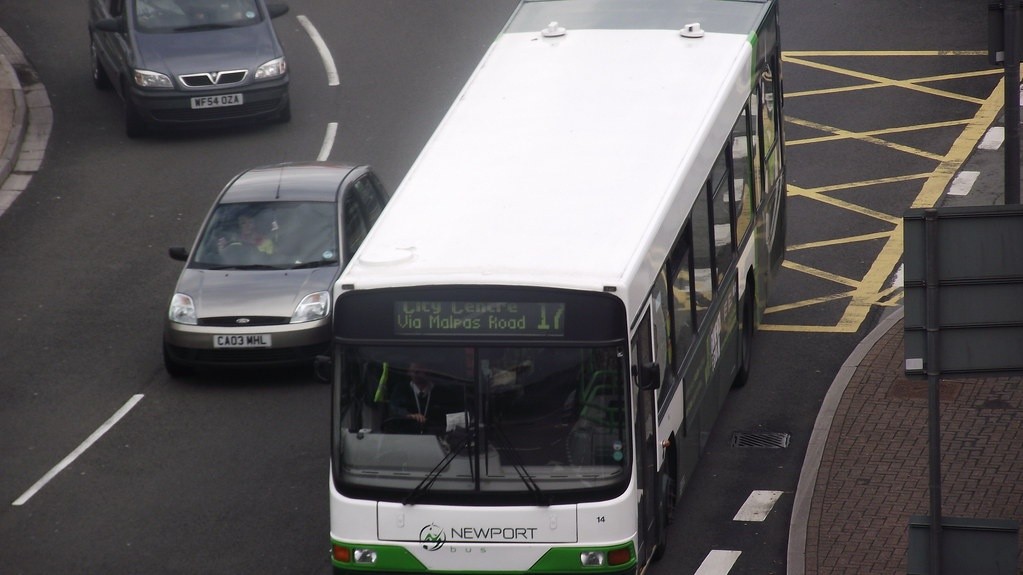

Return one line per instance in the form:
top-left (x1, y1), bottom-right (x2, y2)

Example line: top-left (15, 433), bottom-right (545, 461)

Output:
top-left (387, 353), bottom-right (458, 437)
top-left (218, 212), bottom-right (272, 259)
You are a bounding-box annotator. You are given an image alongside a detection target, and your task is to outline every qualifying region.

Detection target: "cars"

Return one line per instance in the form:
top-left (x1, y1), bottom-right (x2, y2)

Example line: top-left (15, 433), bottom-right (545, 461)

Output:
top-left (83, 2), bottom-right (306, 137)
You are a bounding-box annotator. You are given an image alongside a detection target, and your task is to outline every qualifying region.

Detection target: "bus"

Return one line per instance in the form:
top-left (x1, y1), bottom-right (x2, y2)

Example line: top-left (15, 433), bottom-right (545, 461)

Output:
top-left (322, 0), bottom-right (785, 574)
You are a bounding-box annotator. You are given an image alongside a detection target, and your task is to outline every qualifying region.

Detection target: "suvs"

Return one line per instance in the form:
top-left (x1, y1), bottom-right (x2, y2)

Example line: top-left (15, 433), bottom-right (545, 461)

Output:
top-left (157, 161), bottom-right (386, 378)
